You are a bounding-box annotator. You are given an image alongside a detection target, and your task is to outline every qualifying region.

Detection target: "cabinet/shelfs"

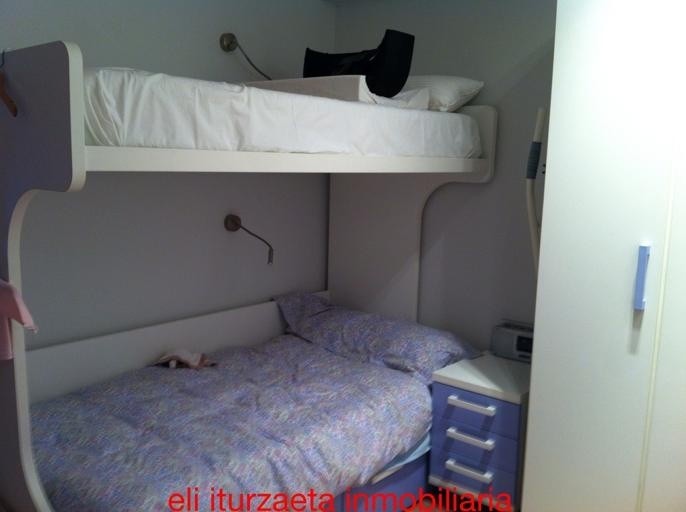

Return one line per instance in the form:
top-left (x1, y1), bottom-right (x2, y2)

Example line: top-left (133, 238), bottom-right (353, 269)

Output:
top-left (522, 1), bottom-right (686, 511)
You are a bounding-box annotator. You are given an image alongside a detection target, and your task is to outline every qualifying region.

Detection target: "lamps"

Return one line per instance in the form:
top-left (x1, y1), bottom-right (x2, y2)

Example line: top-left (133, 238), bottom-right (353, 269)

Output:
top-left (226, 215), bottom-right (273, 266)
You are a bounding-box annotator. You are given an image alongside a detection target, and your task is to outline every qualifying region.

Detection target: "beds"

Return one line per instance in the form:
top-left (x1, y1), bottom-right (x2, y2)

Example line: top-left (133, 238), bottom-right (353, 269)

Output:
top-left (0, 39), bottom-right (499, 512)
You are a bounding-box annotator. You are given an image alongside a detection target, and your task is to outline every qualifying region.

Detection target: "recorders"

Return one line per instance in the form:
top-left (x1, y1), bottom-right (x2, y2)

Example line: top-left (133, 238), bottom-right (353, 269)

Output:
top-left (489, 324), bottom-right (533, 364)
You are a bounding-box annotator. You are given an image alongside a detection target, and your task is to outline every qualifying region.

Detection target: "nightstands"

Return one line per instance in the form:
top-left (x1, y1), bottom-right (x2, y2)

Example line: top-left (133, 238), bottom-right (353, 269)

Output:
top-left (429, 351), bottom-right (531, 512)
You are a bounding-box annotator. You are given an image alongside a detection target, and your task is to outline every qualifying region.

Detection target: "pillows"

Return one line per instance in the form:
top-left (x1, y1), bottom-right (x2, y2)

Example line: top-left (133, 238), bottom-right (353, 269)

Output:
top-left (394, 74), bottom-right (485, 113)
top-left (287, 307), bottom-right (480, 383)
